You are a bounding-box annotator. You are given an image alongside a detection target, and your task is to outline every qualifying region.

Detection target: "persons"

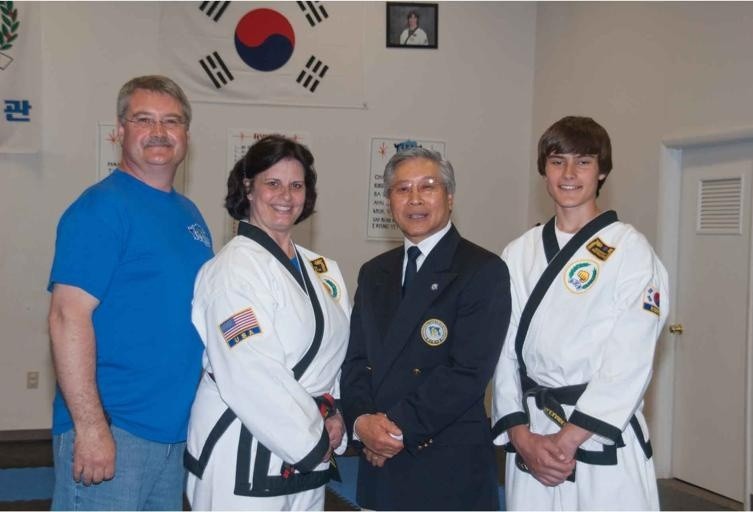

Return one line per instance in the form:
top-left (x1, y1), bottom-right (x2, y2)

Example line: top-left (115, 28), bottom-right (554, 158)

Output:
top-left (338, 143), bottom-right (513, 511)
top-left (185, 130), bottom-right (356, 511)
top-left (45, 75), bottom-right (217, 511)
top-left (489, 113), bottom-right (672, 510)
top-left (399, 10), bottom-right (429, 47)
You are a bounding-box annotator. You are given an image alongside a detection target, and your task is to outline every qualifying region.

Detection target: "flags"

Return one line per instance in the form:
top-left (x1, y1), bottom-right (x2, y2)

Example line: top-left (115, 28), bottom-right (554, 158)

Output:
top-left (157, 1), bottom-right (368, 109)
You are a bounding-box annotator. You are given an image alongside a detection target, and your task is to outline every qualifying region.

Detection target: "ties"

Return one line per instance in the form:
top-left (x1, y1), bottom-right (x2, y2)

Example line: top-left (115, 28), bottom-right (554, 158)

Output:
top-left (403, 246), bottom-right (423, 288)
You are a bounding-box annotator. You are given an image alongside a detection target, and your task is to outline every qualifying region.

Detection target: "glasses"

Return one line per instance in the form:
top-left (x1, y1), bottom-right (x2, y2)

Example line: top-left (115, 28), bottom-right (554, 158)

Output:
top-left (125, 117), bottom-right (186, 129)
top-left (391, 179), bottom-right (448, 195)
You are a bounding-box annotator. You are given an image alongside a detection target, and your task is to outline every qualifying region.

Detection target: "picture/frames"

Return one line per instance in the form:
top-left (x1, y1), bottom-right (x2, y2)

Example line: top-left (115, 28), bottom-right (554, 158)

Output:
top-left (386, 1), bottom-right (439, 48)
